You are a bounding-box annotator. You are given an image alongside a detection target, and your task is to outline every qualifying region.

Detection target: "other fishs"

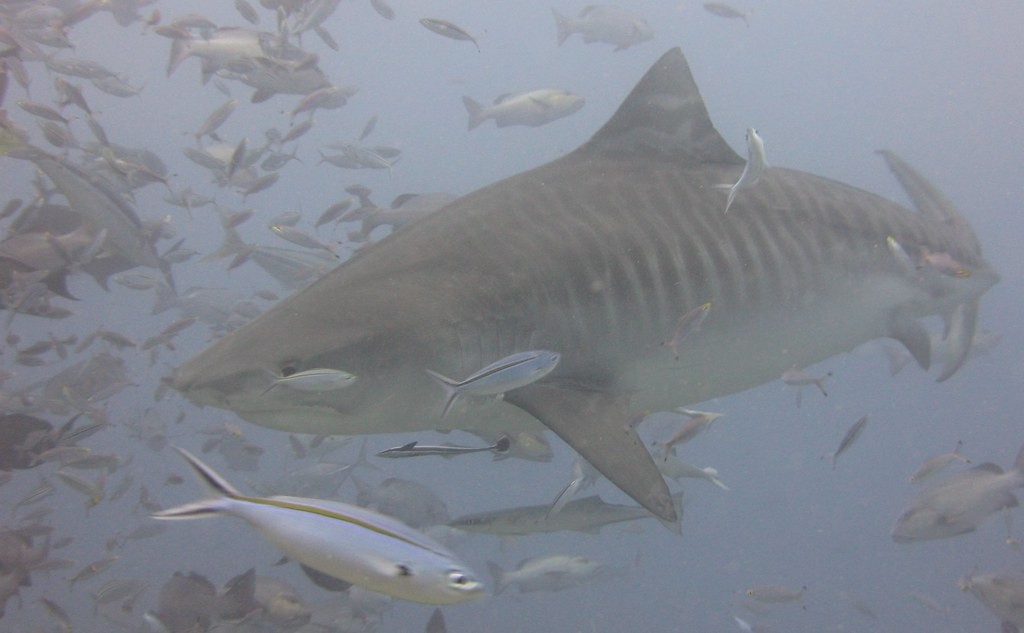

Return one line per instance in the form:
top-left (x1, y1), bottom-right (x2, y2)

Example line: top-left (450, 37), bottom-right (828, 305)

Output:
top-left (781, 236), bottom-right (1024, 632)
top-left (0, 0), bottom-right (765, 633)
